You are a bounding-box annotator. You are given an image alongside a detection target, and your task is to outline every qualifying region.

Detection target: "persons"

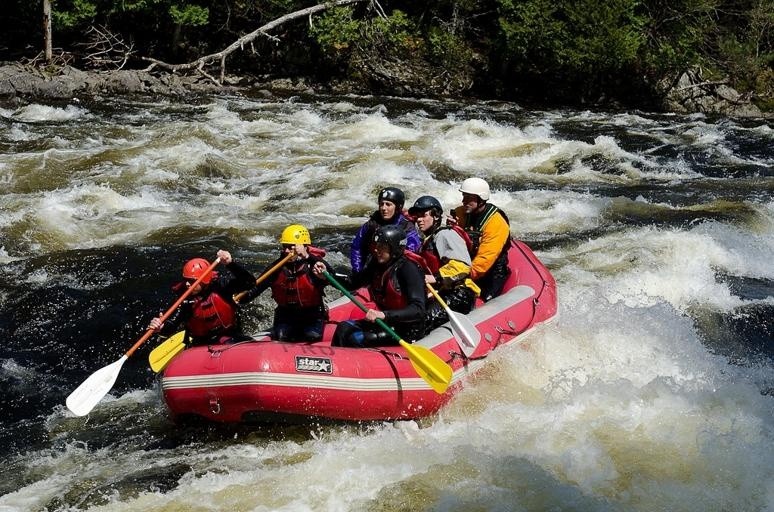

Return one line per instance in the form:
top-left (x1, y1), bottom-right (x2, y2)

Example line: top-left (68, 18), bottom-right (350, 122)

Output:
top-left (146, 250), bottom-right (256, 347)
top-left (446, 177), bottom-right (511, 302)
top-left (407, 196), bottom-right (474, 334)
top-left (350, 187), bottom-right (421, 290)
top-left (235, 224), bottom-right (336, 342)
top-left (313, 225), bottom-right (428, 348)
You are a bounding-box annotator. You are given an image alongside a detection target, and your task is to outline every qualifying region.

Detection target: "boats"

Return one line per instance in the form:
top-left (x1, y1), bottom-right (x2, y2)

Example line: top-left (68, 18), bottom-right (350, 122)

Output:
top-left (152, 232), bottom-right (558, 430)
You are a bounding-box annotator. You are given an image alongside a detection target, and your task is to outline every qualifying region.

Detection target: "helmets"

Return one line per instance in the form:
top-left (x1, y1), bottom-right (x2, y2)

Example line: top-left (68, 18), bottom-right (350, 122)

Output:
top-left (371, 224), bottom-right (408, 261)
top-left (378, 187), bottom-right (405, 209)
top-left (182, 257), bottom-right (213, 286)
top-left (458, 176), bottom-right (491, 201)
top-left (279, 223), bottom-right (312, 246)
top-left (408, 194), bottom-right (444, 216)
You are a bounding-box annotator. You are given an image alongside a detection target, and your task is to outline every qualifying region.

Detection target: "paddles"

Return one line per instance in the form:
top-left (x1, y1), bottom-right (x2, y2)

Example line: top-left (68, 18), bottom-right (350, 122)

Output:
top-left (149, 252), bottom-right (294, 371)
top-left (66, 248), bottom-right (227, 417)
top-left (324, 273), bottom-right (453, 393)
top-left (426, 284), bottom-right (482, 357)
